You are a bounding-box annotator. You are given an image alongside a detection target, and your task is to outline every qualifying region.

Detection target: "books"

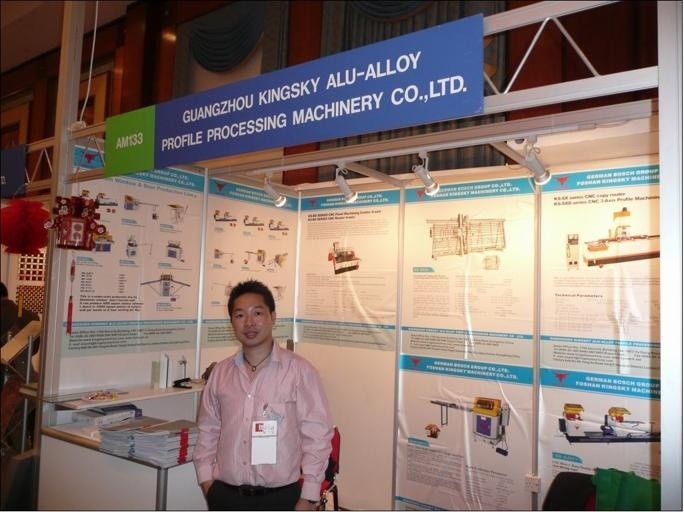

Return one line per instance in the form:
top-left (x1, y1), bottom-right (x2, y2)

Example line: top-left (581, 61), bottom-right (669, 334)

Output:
top-left (98, 416), bottom-right (197, 468)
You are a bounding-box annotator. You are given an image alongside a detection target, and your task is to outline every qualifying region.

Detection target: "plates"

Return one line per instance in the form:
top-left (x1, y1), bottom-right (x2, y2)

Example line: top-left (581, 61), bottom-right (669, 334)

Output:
top-left (81, 395), bottom-right (122, 405)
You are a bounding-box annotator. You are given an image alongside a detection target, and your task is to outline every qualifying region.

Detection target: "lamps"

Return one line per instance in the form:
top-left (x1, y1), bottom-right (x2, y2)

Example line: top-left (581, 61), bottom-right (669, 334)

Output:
top-left (245, 124), bottom-right (598, 207)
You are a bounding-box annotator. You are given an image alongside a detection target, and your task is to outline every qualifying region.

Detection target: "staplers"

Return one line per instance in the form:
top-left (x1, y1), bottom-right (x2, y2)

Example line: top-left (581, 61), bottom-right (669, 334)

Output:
top-left (172, 378), bottom-right (193, 389)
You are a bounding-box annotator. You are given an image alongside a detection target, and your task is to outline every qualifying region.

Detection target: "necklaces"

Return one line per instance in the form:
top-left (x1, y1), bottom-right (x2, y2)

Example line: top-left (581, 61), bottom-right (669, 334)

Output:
top-left (242, 350), bottom-right (271, 373)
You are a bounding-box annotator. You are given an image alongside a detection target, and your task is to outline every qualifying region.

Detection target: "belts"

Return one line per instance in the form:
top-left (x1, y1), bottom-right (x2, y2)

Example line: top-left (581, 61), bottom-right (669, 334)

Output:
top-left (217, 480), bottom-right (299, 497)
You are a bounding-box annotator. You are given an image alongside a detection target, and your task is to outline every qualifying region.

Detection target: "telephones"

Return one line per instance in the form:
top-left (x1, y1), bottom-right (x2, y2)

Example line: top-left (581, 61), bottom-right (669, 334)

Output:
top-left (202, 361), bottom-right (217, 379)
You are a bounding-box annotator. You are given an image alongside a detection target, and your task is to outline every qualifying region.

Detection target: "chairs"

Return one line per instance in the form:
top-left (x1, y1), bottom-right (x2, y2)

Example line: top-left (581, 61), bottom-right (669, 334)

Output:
top-left (298, 424), bottom-right (340, 511)
top-left (539, 473), bottom-right (595, 510)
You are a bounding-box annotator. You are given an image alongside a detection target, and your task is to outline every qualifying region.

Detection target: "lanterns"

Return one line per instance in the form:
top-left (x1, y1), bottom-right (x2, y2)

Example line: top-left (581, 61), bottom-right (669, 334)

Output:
top-left (0, 198), bottom-right (50, 256)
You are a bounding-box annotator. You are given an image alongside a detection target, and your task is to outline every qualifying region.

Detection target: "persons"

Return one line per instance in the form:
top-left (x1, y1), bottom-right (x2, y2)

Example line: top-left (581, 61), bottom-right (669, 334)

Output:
top-left (1, 281), bottom-right (27, 347)
top-left (190, 276), bottom-right (336, 511)
